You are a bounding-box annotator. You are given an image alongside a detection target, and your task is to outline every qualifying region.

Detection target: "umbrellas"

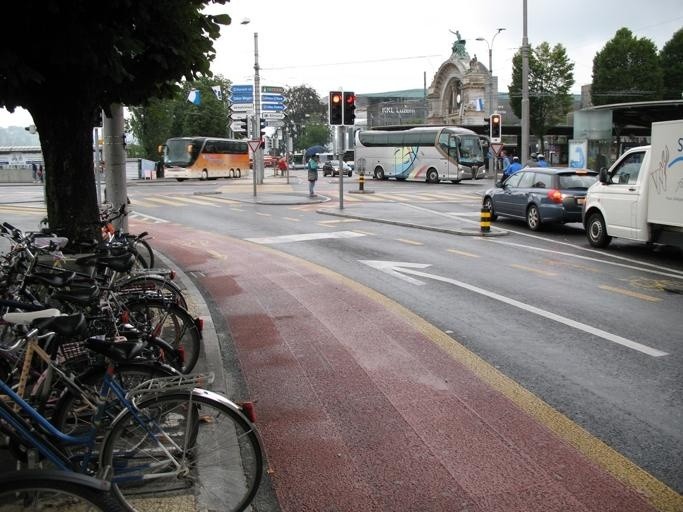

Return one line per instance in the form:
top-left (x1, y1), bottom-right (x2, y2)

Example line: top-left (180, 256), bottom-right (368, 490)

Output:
top-left (303, 145), bottom-right (332, 159)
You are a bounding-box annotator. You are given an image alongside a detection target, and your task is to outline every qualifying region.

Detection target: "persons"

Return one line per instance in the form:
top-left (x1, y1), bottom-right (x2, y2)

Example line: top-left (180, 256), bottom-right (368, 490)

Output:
top-left (305, 153), bottom-right (319, 197)
top-left (278, 155), bottom-right (288, 177)
top-left (38, 164), bottom-right (43, 183)
top-left (524, 152), bottom-right (537, 168)
top-left (31, 162), bottom-right (37, 183)
top-left (155, 161), bottom-right (162, 181)
top-left (467, 54), bottom-right (477, 67)
top-left (506, 155), bottom-right (522, 177)
top-left (536, 152), bottom-right (550, 167)
top-left (449, 30), bottom-right (462, 46)
top-left (500, 150), bottom-right (511, 183)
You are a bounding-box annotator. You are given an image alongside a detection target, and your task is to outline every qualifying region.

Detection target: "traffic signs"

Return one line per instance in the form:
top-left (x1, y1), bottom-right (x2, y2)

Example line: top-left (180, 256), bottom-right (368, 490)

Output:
top-left (260, 85), bottom-right (285, 121)
top-left (224, 84), bottom-right (254, 133)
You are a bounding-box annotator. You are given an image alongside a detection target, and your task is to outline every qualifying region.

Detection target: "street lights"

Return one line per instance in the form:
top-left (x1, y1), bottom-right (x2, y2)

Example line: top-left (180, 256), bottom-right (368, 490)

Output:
top-left (474, 26), bottom-right (508, 113)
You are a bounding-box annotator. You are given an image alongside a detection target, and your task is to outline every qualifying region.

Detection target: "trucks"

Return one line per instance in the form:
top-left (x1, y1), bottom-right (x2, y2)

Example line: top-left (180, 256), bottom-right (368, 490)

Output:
top-left (582, 120), bottom-right (683, 249)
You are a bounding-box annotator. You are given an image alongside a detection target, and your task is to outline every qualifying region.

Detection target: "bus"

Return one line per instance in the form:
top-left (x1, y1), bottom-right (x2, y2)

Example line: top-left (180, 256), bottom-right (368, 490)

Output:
top-left (291, 153), bottom-right (334, 170)
top-left (353, 127), bottom-right (486, 184)
top-left (157, 137), bottom-right (249, 182)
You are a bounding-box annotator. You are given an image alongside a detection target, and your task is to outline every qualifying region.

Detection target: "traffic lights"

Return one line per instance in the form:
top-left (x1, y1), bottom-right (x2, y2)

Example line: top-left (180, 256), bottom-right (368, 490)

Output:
top-left (328, 91), bottom-right (355, 128)
top-left (482, 114), bottom-right (503, 144)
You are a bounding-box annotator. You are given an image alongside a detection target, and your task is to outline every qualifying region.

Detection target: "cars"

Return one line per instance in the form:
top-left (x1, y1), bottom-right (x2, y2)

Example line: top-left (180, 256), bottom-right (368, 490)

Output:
top-left (481, 167), bottom-right (600, 231)
top-left (322, 160), bottom-right (352, 177)
top-left (248, 156), bottom-right (281, 170)
